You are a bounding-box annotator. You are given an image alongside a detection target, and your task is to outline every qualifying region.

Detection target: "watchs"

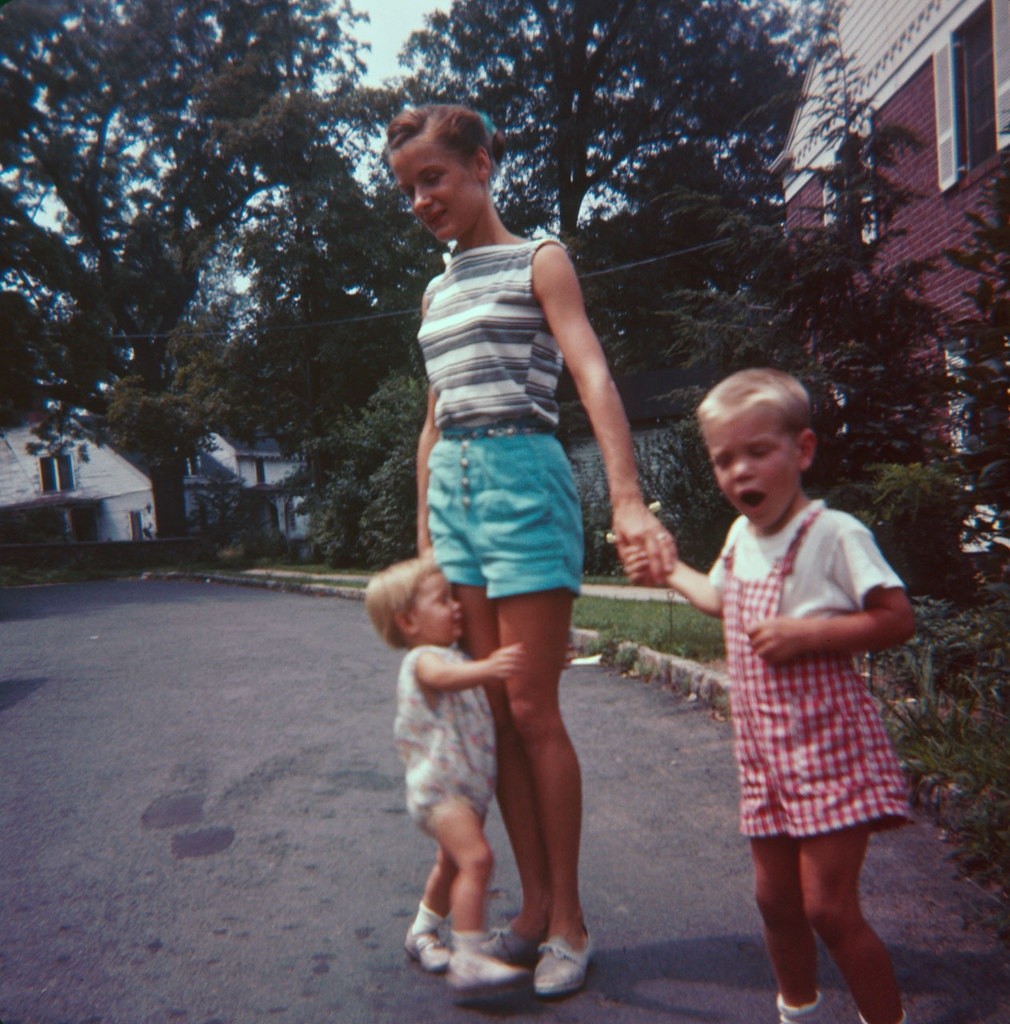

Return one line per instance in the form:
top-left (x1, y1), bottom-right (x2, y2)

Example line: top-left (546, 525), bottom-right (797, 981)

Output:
top-left (362, 559), bottom-right (528, 1003)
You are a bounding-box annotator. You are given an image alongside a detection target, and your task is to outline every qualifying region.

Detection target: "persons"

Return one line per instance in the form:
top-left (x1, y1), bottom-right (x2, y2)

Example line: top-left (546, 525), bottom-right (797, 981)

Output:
top-left (380, 103), bottom-right (678, 995)
top-left (619, 368), bottom-right (923, 1024)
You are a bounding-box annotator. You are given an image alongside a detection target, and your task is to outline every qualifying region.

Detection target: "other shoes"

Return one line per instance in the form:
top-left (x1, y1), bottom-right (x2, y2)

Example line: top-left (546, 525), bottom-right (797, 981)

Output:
top-left (534, 922), bottom-right (594, 995)
top-left (400, 924), bottom-right (453, 972)
top-left (448, 959), bottom-right (530, 999)
top-left (483, 926), bottom-right (539, 965)
